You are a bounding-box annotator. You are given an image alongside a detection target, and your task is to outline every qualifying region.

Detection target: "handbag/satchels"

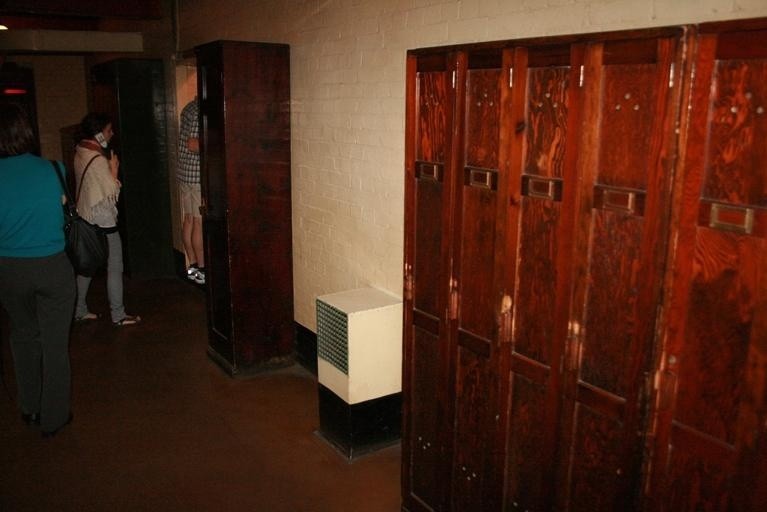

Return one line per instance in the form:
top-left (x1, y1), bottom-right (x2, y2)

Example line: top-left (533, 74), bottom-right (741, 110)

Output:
top-left (64, 210), bottom-right (109, 279)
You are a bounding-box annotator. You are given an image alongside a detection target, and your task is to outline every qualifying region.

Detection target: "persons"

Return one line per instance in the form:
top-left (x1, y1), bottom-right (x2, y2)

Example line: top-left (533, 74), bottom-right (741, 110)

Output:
top-left (0, 99), bottom-right (75, 443)
top-left (176, 96), bottom-right (212, 287)
top-left (64, 111), bottom-right (143, 327)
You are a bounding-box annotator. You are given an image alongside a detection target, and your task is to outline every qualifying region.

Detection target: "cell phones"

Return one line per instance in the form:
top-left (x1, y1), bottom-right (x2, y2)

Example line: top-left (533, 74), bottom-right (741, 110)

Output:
top-left (95, 132), bottom-right (118, 160)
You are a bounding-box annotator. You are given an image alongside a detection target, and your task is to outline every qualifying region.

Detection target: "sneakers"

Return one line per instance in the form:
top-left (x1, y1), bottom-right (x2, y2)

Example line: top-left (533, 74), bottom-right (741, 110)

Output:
top-left (21, 408), bottom-right (72, 439)
top-left (187, 263), bottom-right (206, 285)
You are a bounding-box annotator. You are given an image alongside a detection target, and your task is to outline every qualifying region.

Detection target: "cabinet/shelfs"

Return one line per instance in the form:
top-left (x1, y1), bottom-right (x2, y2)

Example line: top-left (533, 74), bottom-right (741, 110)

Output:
top-left (400, 16), bottom-right (767, 512)
top-left (193, 39), bottom-right (295, 379)
top-left (87, 58), bottom-right (176, 280)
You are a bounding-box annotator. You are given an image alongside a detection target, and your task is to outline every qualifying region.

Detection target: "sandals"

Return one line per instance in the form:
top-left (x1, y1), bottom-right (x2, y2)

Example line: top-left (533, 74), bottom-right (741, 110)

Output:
top-left (113, 312), bottom-right (144, 330)
top-left (73, 311), bottom-right (104, 322)
